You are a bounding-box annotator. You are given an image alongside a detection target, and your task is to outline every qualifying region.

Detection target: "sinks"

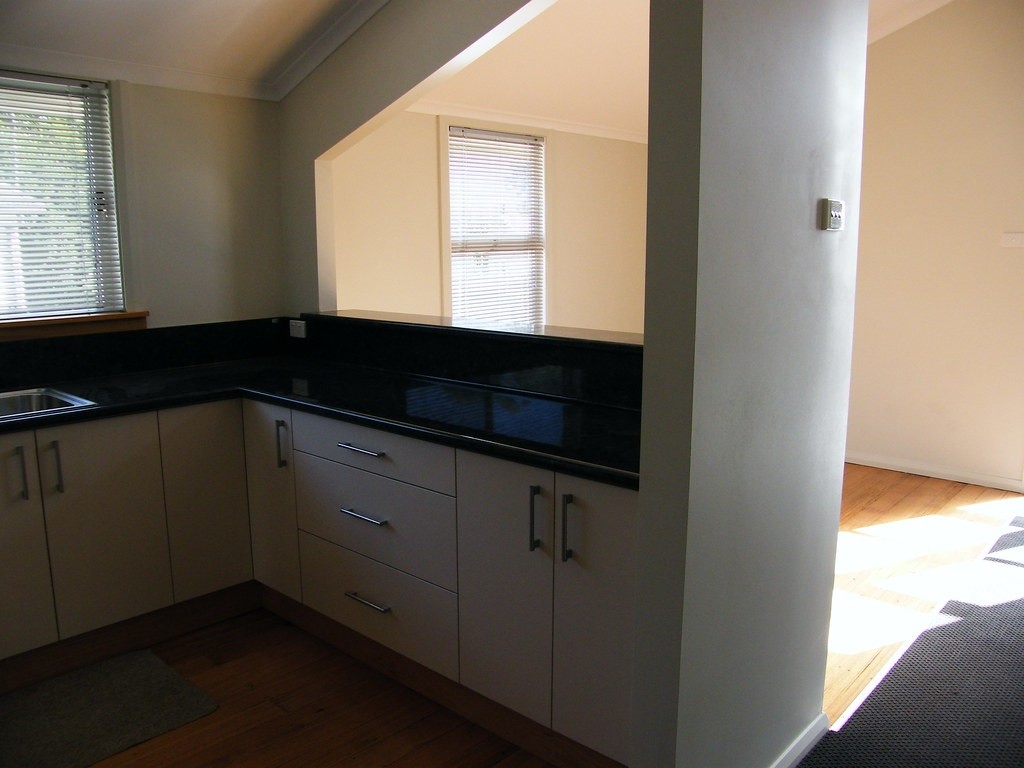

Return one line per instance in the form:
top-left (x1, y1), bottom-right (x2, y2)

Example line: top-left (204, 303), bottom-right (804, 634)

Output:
top-left (0, 387), bottom-right (99, 423)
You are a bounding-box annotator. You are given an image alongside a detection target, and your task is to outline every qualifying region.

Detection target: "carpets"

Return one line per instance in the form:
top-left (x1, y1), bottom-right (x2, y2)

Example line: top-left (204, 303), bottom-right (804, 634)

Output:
top-left (794, 512), bottom-right (1024, 768)
top-left (0, 646), bottom-right (219, 768)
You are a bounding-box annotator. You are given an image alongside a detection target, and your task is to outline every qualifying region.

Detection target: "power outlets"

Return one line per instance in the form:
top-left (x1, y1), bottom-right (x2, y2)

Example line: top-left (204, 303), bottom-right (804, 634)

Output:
top-left (289, 319), bottom-right (307, 339)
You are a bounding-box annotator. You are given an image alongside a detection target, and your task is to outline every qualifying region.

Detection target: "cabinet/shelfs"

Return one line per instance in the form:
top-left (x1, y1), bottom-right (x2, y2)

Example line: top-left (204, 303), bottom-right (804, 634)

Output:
top-left (457, 449), bottom-right (638, 768)
top-left (243, 398), bottom-right (300, 624)
top-left (0, 411), bottom-right (177, 696)
top-left (291, 409), bottom-right (457, 718)
